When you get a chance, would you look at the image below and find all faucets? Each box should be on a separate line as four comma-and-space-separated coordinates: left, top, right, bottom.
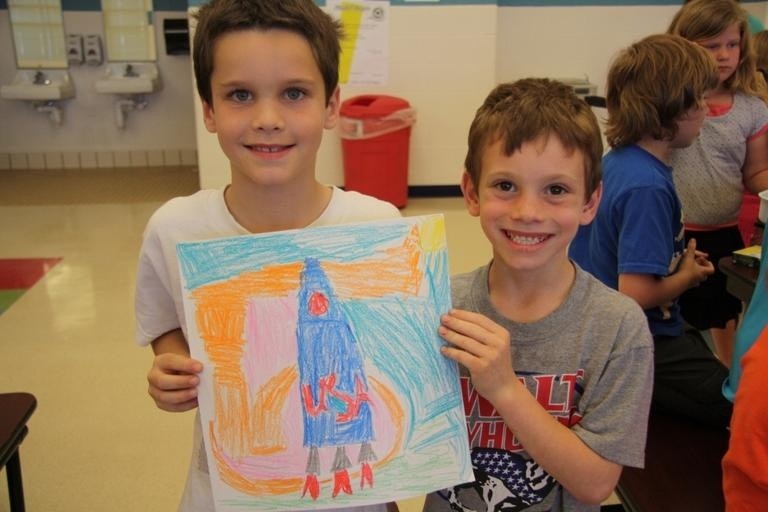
122, 63, 137, 76
32, 72, 43, 84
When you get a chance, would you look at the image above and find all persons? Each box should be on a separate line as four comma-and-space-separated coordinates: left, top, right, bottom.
419, 78, 655, 512
568, 32, 730, 497
662, 2, 767, 364
133, 2, 408, 512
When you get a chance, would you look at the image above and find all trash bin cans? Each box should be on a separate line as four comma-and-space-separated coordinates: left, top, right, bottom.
339, 95, 416, 209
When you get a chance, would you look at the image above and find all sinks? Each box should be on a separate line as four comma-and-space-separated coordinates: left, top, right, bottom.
95, 62, 163, 96
0, 70, 74, 102
